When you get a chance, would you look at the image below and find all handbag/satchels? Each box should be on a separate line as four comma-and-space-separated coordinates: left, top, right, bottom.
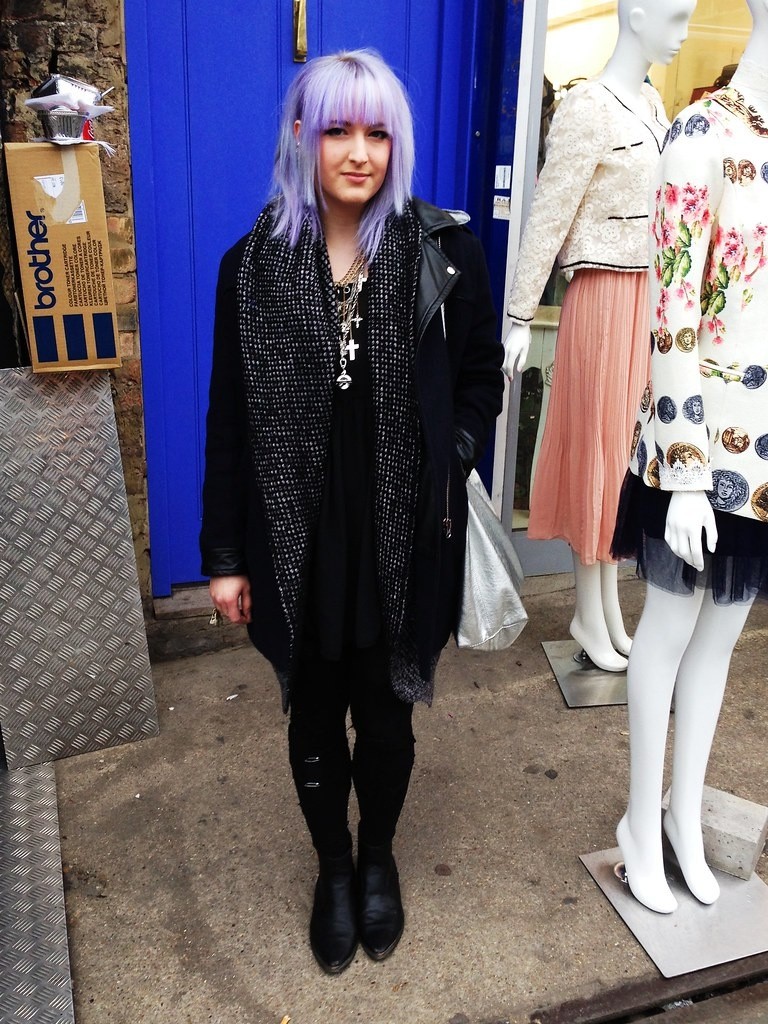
455, 470, 529, 655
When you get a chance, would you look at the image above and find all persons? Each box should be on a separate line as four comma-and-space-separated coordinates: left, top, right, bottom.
199, 48, 505, 975
502, 0, 697, 672
616, 0, 768, 914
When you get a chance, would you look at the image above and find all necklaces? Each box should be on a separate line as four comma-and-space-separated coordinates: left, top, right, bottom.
334, 249, 369, 391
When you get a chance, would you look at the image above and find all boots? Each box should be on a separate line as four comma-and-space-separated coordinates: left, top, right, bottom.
289, 724, 358, 974
350, 730, 415, 961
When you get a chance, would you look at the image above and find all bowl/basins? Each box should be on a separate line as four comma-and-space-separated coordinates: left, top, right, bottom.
33, 73, 101, 105
37, 109, 90, 141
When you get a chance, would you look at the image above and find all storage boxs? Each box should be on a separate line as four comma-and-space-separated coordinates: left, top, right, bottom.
4, 139, 124, 373
